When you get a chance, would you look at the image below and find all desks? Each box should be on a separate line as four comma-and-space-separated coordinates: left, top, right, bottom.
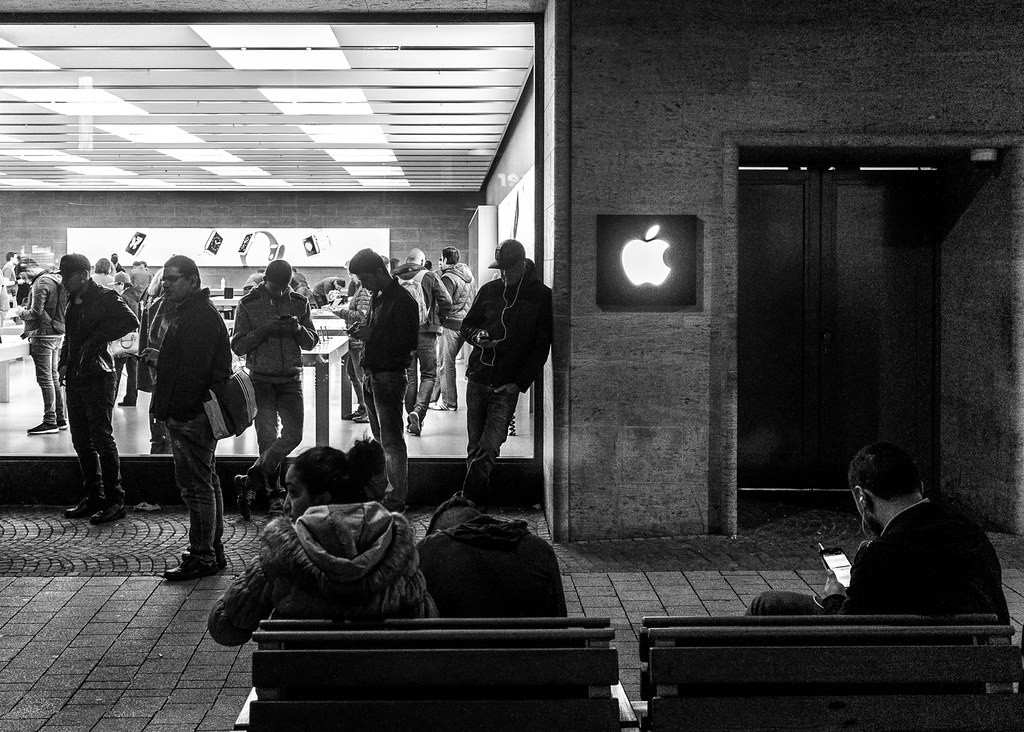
0, 289, 353, 448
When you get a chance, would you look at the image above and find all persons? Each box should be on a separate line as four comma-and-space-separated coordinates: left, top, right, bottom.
141, 255, 233, 581
349, 248, 420, 515
91, 253, 181, 454
0, 252, 67, 435
231, 260, 319, 521
328, 246, 477, 436
49, 253, 140, 524
208, 438, 438, 648
461, 239, 553, 513
744, 439, 1011, 627
243, 266, 345, 309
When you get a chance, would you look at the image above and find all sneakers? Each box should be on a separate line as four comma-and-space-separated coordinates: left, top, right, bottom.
57, 421, 68, 429
182, 551, 227, 567
428, 402, 437, 408
90, 504, 127, 525
234, 474, 255, 520
27, 423, 59, 434
433, 404, 456, 411
267, 498, 285, 521
164, 557, 219, 580
352, 413, 370, 423
65, 498, 107, 518
345, 409, 366, 419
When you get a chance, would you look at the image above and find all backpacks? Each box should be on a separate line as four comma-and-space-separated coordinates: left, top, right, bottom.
395, 270, 432, 325
35, 275, 67, 333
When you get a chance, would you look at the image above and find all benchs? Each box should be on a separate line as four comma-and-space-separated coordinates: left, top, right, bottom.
630, 611, 1024, 732
233, 615, 639, 732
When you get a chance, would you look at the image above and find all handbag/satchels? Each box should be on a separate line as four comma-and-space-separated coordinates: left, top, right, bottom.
107, 332, 139, 358
202, 370, 259, 440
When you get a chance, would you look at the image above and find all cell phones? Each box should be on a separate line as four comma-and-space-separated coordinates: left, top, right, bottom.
820, 548, 852, 590
125, 351, 153, 363
479, 336, 492, 341
343, 321, 359, 333
279, 314, 292, 320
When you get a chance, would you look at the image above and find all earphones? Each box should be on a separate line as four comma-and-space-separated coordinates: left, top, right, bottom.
859, 497, 866, 510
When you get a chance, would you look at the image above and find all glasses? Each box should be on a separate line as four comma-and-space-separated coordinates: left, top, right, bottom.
270, 285, 290, 292
162, 273, 184, 282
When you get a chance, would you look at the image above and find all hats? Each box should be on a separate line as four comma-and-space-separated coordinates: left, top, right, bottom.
49, 253, 91, 274
488, 239, 525, 269
107, 271, 131, 285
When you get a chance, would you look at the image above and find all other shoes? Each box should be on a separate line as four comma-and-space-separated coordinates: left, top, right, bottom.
409, 412, 421, 436
381, 502, 403, 513
118, 402, 137, 408
